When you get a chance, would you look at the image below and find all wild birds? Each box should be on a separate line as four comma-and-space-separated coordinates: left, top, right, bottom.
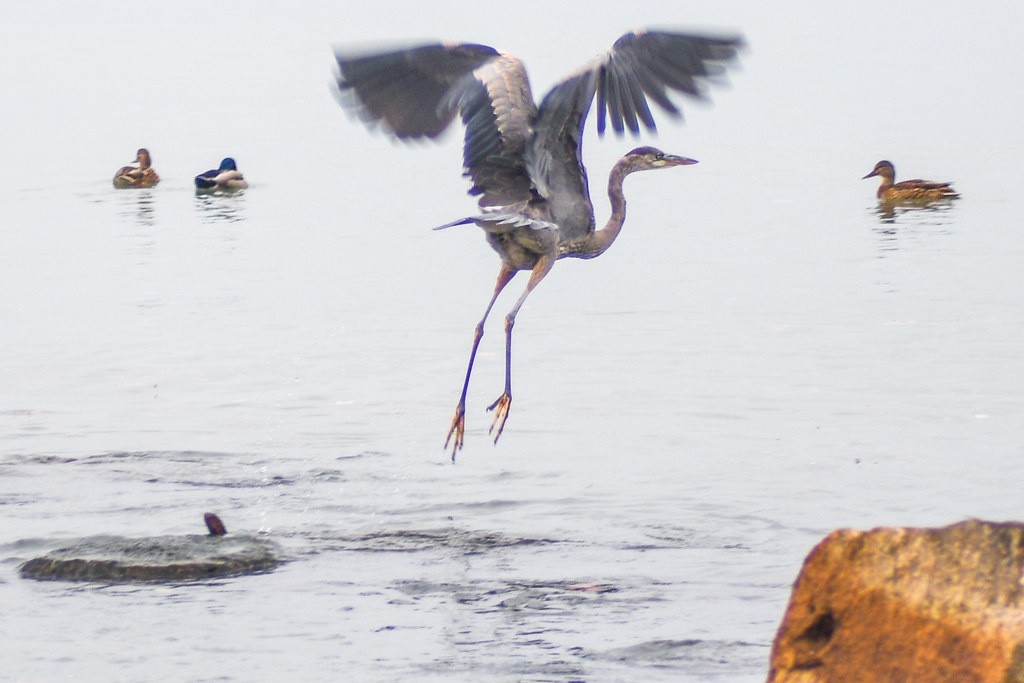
317, 23, 755, 465
194, 157, 249, 196
861, 159, 962, 204
112, 148, 160, 190
